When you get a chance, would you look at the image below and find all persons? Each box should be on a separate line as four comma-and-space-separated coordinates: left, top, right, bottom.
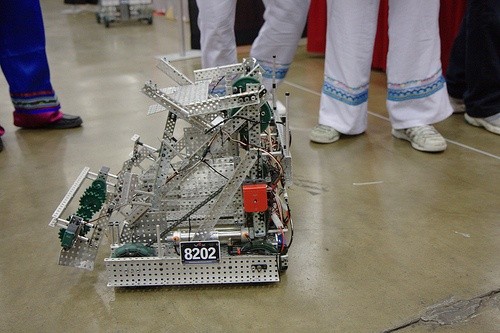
309, 0, 453, 152
0, 0, 82, 151
195, 0, 310, 125
445, 0, 500, 135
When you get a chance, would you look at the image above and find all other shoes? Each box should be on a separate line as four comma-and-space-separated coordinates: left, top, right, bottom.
22, 112, 83, 130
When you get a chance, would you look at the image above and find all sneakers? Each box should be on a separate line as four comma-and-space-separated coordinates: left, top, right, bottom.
449, 96, 465, 113
309, 124, 340, 144
464, 112, 500, 135
391, 124, 447, 153
